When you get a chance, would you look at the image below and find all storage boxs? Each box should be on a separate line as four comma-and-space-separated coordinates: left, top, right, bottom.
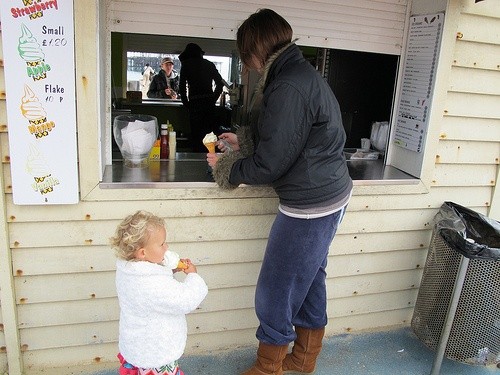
343, 147, 380, 161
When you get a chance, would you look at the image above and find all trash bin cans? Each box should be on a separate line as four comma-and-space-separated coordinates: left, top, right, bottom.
410, 201, 500, 367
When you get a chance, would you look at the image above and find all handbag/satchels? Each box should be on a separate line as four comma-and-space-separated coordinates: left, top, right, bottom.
142, 72, 144, 75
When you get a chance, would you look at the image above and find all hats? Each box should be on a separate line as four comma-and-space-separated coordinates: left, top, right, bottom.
161, 57, 174, 64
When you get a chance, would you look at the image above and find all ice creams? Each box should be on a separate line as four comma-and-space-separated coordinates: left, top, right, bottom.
202, 131, 217, 153
163, 250, 188, 269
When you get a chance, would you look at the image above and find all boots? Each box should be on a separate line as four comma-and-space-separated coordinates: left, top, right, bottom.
282, 326, 325, 374
240, 342, 289, 375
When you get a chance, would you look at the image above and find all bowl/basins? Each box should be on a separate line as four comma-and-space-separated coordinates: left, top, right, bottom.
113, 114, 158, 169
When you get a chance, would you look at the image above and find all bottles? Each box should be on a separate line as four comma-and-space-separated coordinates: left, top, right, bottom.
161, 120, 176, 160
220, 91, 226, 107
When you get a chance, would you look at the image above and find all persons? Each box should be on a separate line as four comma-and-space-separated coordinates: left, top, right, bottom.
206, 9, 355, 375
110, 210, 208, 375
178, 43, 225, 152
147, 57, 180, 146
142, 63, 155, 87
